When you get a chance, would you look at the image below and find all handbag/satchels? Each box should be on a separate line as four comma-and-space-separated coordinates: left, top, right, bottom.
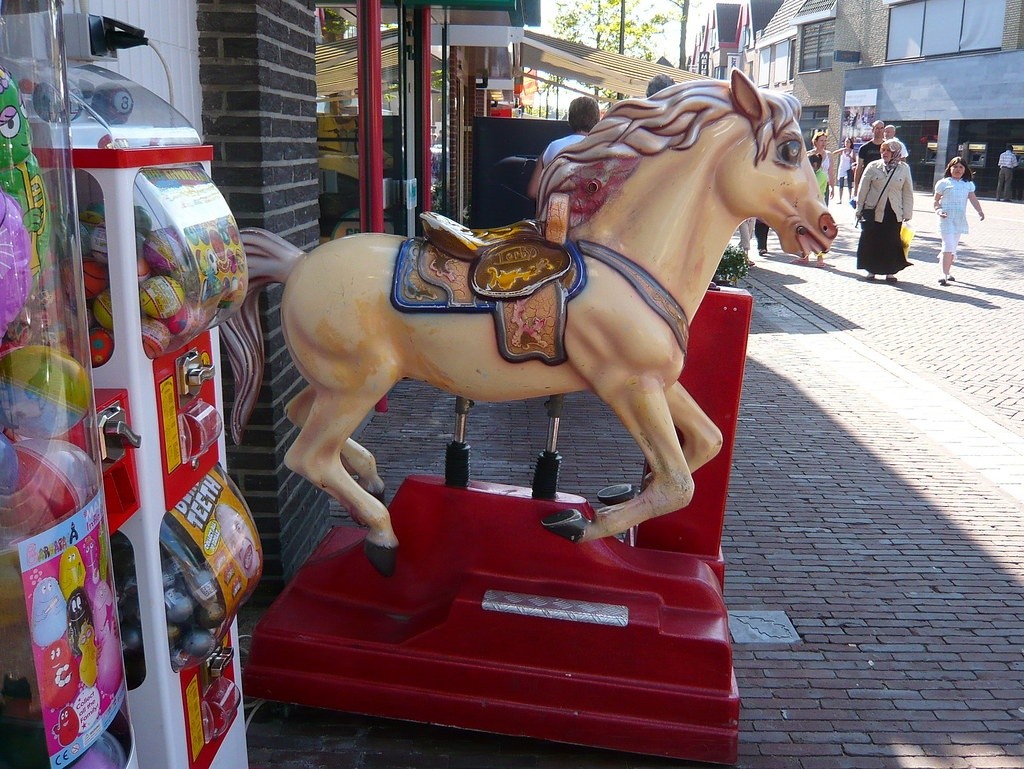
860, 209, 875, 231
847, 169, 853, 183
900, 219, 916, 262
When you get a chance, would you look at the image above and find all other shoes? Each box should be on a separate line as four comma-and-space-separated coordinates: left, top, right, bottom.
866, 275, 874, 280
790, 257, 808, 265
759, 249, 766, 256
997, 198, 1000, 200
748, 260, 754, 266
947, 276, 954, 281
939, 279, 946, 285
1003, 198, 1012, 202
886, 276, 897, 282
816, 262, 825, 268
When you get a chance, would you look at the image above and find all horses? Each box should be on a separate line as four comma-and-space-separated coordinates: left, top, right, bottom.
219, 66, 838, 579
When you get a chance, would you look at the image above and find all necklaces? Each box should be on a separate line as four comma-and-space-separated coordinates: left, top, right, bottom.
854, 139, 913, 282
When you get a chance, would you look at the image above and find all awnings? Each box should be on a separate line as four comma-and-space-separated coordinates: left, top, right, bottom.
515, 29, 720, 104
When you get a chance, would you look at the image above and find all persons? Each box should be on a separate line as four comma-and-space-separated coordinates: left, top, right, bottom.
529, 96, 601, 192
805, 132, 835, 207
854, 120, 887, 197
790, 153, 827, 267
993, 144, 1018, 201
933, 156, 984, 283
884, 125, 908, 163
754, 216, 769, 256
739, 217, 757, 267
833, 136, 855, 204
